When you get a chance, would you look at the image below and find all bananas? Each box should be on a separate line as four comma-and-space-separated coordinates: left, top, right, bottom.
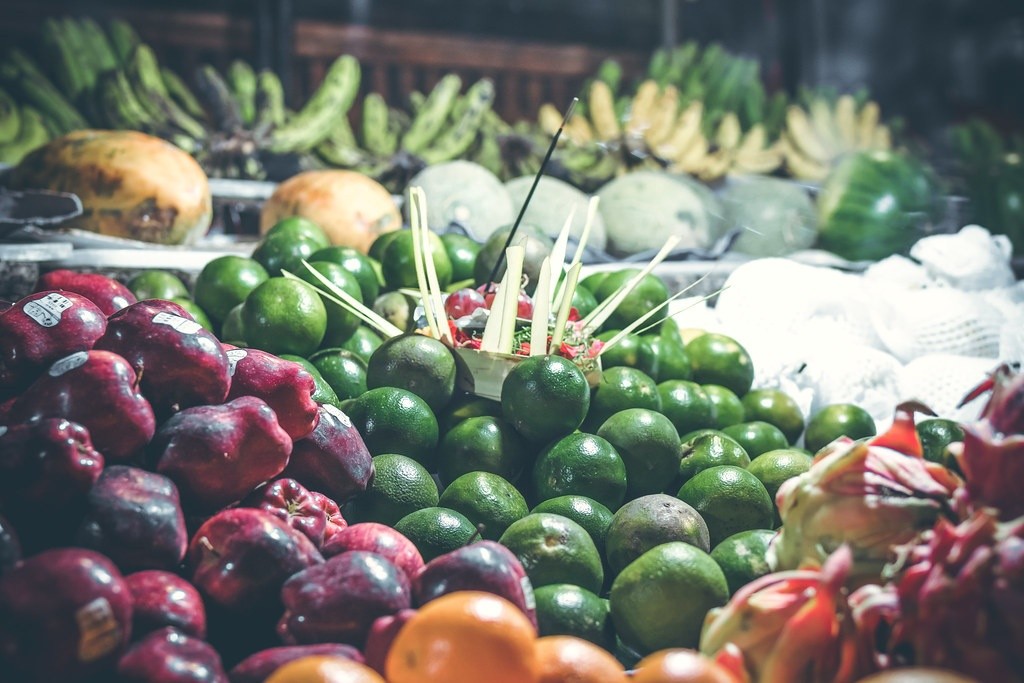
0, 0, 1024, 192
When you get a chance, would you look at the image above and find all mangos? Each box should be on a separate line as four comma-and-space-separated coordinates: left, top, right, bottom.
17, 132, 818, 264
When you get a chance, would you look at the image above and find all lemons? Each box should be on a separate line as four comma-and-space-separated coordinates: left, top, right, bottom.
128, 219, 975, 656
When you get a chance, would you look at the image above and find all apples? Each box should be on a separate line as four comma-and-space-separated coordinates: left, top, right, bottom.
0, 267, 537, 683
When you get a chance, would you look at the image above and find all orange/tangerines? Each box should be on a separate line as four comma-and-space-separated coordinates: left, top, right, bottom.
266, 590, 743, 683
